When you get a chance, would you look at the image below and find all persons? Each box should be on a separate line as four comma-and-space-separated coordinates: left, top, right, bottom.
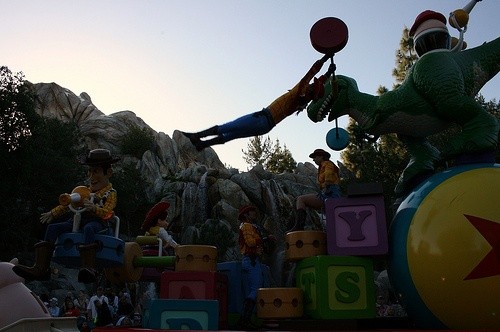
142, 201, 180, 257
376, 295, 407, 318
284, 149, 342, 232
12, 148, 117, 283
183, 53, 336, 151
40, 286, 141, 332
237, 203, 277, 322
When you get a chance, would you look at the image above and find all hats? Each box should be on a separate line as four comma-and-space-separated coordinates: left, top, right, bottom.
49, 297, 59, 305
